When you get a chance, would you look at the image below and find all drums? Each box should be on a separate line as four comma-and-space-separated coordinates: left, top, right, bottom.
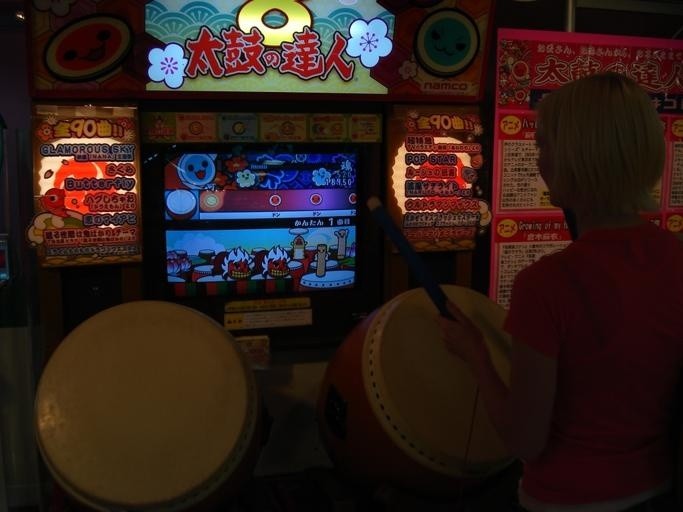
35, 301, 264, 511
318, 286, 516, 493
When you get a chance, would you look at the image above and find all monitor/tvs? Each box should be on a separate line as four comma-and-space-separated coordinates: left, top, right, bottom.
153, 142, 358, 298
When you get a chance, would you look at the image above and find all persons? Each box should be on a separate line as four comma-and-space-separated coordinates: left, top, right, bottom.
434, 72, 683, 510
